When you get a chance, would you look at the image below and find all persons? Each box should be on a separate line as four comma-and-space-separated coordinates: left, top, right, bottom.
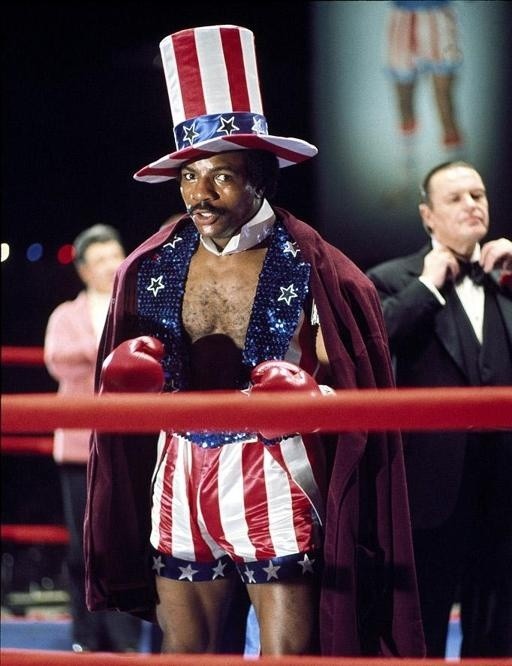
45, 223, 147, 658
380, 1, 471, 198
365, 159, 512, 659
80, 147, 427, 661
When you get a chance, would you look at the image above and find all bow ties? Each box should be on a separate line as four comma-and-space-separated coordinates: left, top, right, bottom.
447, 256, 492, 290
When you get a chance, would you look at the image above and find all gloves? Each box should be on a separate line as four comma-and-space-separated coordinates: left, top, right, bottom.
248, 360, 339, 441
100, 333, 166, 397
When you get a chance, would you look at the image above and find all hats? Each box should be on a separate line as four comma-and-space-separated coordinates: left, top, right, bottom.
131, 20, 320, 183
